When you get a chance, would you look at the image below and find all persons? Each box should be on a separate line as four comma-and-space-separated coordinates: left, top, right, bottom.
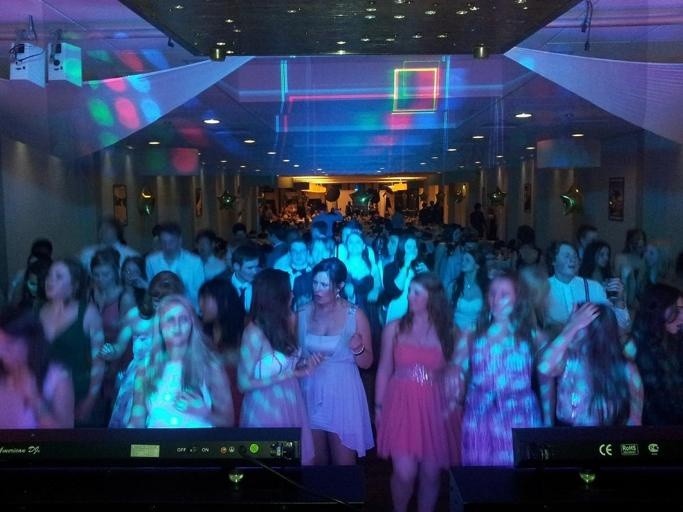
1, 200, 683, 512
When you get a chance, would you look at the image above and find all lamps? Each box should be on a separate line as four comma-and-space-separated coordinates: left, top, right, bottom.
209, 45, 226, 61
471, 40, 490, 59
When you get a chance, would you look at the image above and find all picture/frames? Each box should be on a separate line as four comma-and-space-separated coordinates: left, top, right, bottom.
607, 176, 624, 221
111, 184, 128, 227
